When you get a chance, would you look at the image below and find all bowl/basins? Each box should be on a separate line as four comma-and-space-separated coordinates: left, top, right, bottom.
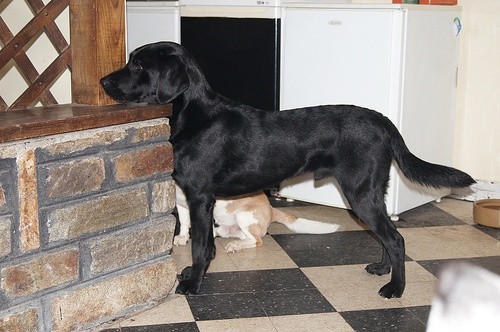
473, 199, 500, 228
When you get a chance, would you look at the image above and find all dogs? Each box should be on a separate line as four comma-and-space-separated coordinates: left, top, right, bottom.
99, 41, 478, 299
173, 181, 341, 254
